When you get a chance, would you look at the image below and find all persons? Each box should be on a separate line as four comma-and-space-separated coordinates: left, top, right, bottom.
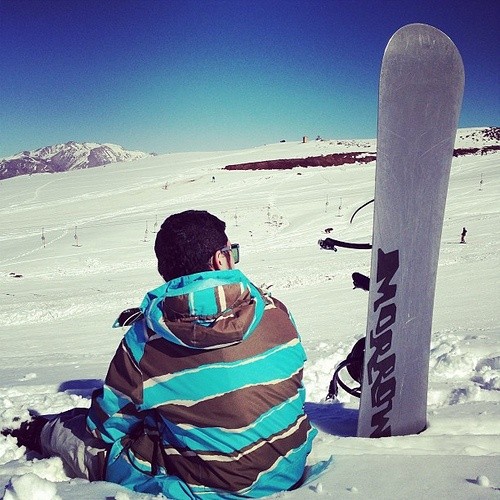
460, 227, 467, 243
1, 210, 318, 500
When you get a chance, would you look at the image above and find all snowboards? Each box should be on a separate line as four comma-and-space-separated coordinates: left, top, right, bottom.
357, 24, 465, 441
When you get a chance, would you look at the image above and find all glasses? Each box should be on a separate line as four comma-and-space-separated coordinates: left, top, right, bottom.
220, 244, 240, 264
